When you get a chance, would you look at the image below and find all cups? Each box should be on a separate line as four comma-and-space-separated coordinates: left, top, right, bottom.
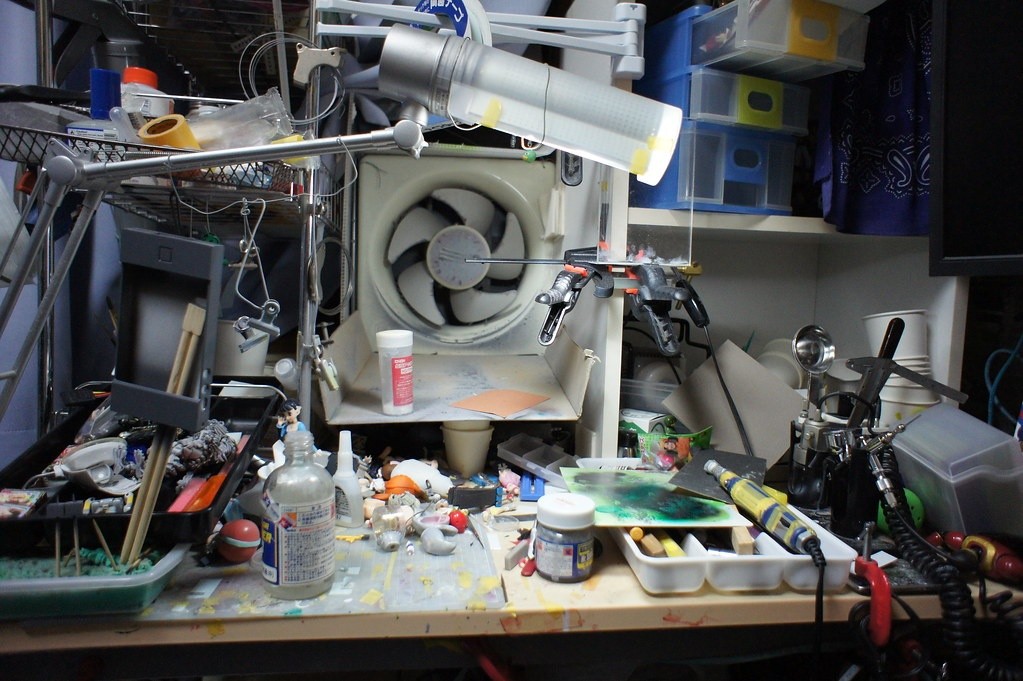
212, 319, 269, 376
441, 426, 495, 478
753, 308, 940, 430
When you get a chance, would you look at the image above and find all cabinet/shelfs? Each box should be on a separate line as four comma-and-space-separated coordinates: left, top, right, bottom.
0, 1, 1023, 681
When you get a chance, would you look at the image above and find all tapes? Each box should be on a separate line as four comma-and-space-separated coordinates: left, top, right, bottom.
139, 113, 200, 152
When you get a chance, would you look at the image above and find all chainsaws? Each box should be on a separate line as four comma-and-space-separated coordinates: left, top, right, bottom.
465, 247, 709, 361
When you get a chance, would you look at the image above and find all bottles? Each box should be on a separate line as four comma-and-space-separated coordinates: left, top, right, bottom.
332, 431, 363, 528
375, 329, 414, 415
119, 66, 175, 117
274, 358, 299, 390
259, 431, 335, 599
533, 493, 595, 582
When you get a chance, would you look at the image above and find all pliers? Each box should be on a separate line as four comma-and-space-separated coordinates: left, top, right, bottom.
537, 271, 581, 347
631, 265, 681, 356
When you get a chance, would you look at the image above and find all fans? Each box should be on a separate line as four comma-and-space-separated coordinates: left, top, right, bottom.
353, 146, 559, 355
